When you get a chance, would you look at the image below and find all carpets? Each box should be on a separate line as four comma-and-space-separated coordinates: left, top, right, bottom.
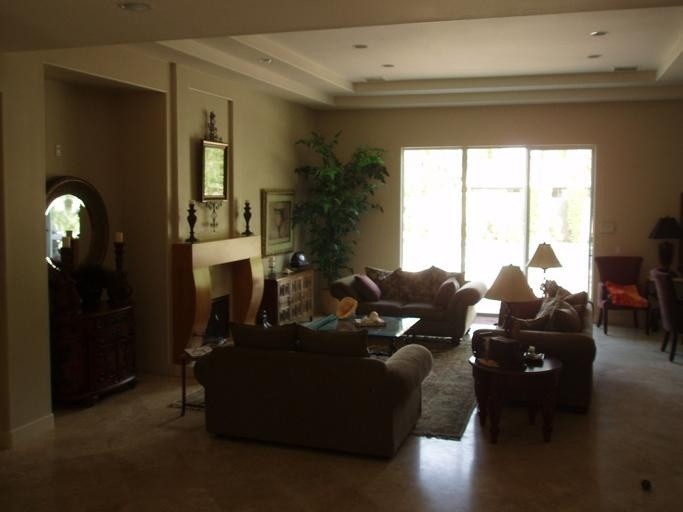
170, 333, 478, 441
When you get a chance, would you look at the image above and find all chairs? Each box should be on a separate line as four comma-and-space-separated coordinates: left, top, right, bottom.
594, 256, 682, 361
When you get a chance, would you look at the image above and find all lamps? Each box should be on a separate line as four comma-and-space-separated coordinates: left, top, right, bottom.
648, 215, 683, 277
484, 242, 562, 364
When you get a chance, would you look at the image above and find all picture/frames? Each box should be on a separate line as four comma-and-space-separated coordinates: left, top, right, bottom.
201, 139, 228, 204
260, 188, 295, 259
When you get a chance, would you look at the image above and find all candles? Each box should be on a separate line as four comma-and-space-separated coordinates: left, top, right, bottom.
245, 200, 249, 203
62, 236, 70, 247
190, 200, 195, 204
116, 231, 123, 242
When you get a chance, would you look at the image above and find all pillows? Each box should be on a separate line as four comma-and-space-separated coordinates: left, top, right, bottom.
354, 266, 465, 311
503, 280, 588, 333
226, 319, 370, 357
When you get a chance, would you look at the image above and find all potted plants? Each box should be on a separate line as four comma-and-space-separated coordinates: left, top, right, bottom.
292, 127, 389, 315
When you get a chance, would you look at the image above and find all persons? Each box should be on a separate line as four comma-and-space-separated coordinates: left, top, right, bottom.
269, 206, 288, 239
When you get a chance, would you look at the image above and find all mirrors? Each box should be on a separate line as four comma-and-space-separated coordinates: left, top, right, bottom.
46, 175, 110, 284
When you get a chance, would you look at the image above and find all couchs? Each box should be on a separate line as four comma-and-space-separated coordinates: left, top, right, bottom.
192, 341, 433, 459
472, 297, 597, 416
329, 271, 484, 343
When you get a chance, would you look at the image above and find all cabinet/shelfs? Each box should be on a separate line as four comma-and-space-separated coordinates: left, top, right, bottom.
257, 267, 314, 326
50, 300, 138, 406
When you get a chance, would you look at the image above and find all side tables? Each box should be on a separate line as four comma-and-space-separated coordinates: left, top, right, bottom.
468, 353, 564, 443
180, 340, 225, 417
